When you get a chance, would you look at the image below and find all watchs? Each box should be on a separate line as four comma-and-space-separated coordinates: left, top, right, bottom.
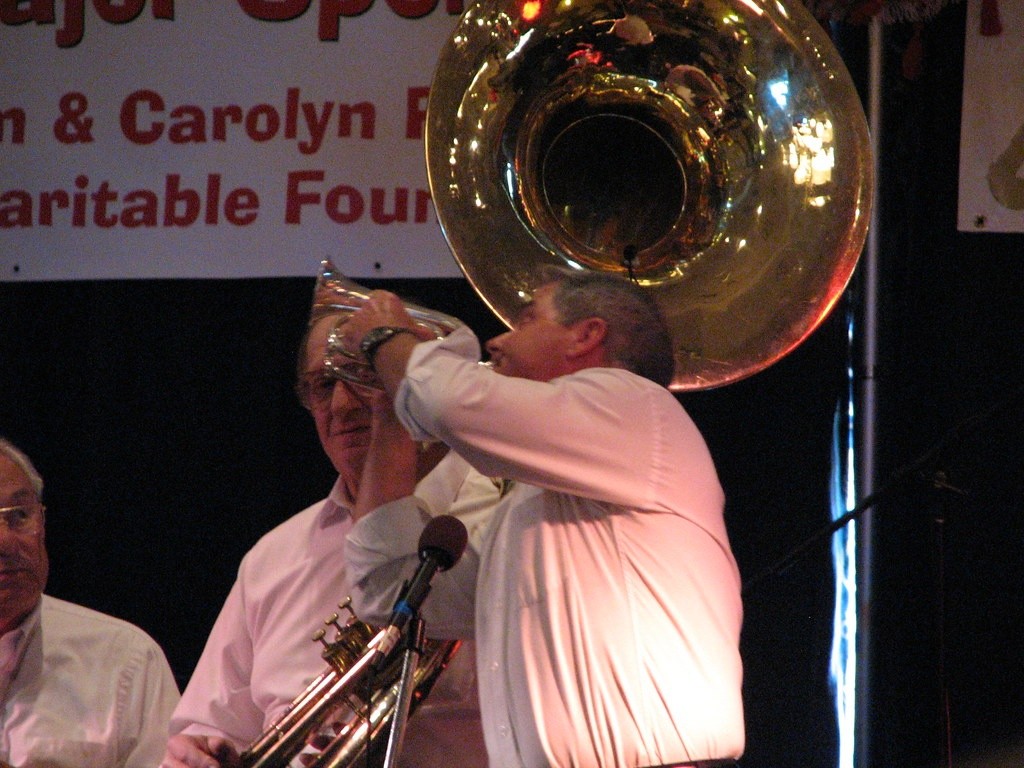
358, 325, 420, 373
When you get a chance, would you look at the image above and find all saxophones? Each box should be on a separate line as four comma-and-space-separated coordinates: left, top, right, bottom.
226, 593, 466, 768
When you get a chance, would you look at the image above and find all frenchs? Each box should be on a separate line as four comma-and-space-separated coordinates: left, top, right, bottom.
304, 1, 877, 391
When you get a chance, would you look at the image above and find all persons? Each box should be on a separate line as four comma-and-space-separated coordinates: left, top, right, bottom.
2, 441, 182, 768
343, 268, 747, 768
155, 280, 504, 768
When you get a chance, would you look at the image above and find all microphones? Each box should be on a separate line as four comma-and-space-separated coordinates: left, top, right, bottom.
371, 515, 468, 669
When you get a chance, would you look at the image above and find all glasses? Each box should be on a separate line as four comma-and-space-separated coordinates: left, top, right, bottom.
296, 359, 391, 410
0, 502, 46, 538
